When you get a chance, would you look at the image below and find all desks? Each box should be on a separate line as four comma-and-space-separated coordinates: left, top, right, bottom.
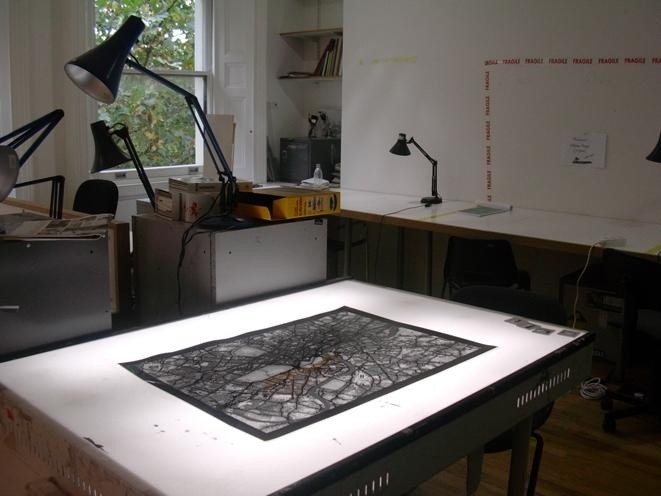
2, 274, 596, 496
132, 213, 329, 326
330, 185, 661, 268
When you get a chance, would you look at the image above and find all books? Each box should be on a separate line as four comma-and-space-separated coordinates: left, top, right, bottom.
278, 34, 344, 77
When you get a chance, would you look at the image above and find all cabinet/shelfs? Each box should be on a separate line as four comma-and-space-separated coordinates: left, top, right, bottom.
277, 27, 343, 81
279, 138, 340, 184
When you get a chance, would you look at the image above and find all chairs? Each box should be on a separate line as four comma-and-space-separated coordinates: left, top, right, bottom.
442, 237, 659, 493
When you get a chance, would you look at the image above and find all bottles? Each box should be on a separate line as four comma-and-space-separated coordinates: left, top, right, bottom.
314, 164, 323, 187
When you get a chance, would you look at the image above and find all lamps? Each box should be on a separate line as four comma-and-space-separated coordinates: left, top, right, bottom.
390, 132, 444, 205
63, 15, 248, 232
0, 108, 65, 220
